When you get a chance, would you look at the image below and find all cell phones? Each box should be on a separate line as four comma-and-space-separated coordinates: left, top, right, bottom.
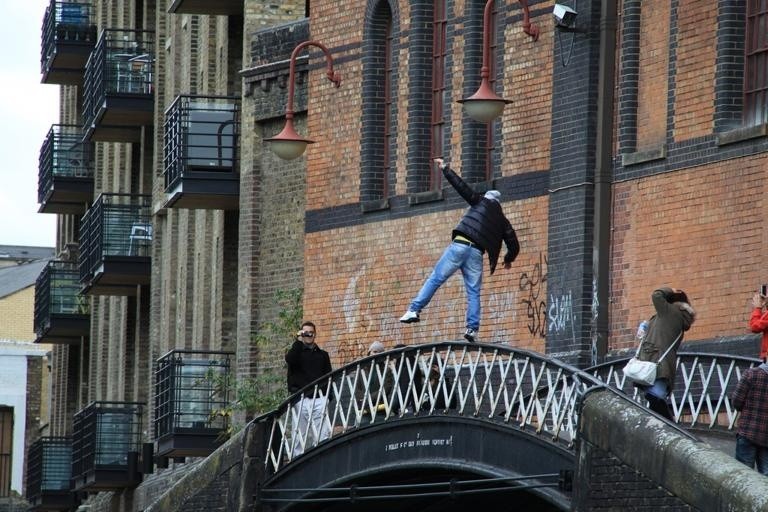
760, 285, 768, 297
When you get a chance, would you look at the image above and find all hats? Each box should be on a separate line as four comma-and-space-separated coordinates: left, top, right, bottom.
484, 189, 501, 202
366, 341, 386, 354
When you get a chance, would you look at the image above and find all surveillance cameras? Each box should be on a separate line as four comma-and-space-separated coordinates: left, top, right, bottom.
552, 3, 578, 28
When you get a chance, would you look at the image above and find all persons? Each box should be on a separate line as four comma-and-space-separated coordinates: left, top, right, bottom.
389, 344, 420, 414
634, 287, 693, 424
730, 357, 767, 478
748, 291, 767, 366
284, 322, 332, 460
399, 157, 520, 344
422, 356, 456, 412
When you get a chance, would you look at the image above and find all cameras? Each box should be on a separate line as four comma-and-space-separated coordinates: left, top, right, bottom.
301, 332, 314, 337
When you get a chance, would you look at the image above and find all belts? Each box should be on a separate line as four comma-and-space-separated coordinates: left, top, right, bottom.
452, 238, 489, 256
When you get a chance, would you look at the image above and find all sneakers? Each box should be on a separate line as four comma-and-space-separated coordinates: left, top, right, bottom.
399, 310, 419, 324
464, 329, 476, 342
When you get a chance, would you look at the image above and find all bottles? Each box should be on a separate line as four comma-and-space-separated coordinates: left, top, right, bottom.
636, 319, 649, 339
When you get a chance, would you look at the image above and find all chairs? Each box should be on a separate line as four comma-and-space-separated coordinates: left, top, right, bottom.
124, 51, 154, 94
125, 219, 155, 257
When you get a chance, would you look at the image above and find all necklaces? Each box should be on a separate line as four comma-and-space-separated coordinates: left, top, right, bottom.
354, 341, 394, 427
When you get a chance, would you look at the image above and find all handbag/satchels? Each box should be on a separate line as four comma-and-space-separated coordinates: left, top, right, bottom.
622, 353, 660, 387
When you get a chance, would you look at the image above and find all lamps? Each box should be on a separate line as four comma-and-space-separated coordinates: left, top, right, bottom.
454, 0, 542, 126
261, 38, 340, 160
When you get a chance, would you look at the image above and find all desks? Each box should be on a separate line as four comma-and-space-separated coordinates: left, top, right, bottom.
112, 48, 148, 95
58, 283, 84, 315
